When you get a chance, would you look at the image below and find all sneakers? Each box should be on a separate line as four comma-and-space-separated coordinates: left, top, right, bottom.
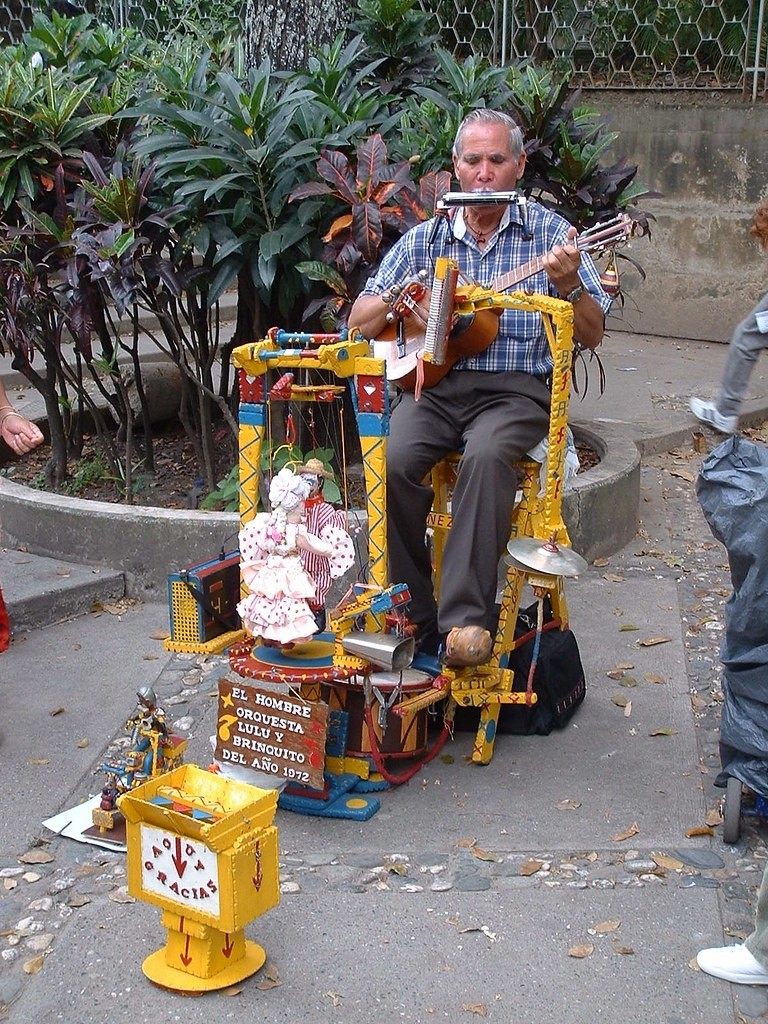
697, 944, 768, 984
689, 396, 738, 434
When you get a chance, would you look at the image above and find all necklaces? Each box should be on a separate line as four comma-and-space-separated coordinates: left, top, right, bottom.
463, 215, 499, 243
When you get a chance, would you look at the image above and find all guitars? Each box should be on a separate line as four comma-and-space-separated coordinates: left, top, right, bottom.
373, 213, 632, 392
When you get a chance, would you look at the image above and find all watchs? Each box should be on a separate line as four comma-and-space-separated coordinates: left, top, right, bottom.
559, 282, 584, 302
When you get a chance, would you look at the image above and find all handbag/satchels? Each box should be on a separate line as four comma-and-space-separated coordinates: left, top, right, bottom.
419, 596, 586, 735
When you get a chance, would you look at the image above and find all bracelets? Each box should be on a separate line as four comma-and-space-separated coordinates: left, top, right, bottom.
0, 405, 16, 412
0, 412, 24, 436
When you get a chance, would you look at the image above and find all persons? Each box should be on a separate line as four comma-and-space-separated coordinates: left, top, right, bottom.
0, 381, 45, 652
347, 108, 613, 668
236, 458, 355, 650
695, 856, 768, 984
689, 197, 768, 435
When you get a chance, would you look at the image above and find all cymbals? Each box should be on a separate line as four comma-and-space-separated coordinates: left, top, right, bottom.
503, 536, 588, 576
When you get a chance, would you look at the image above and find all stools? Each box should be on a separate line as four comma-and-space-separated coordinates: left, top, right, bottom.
430, 450, 569, 765
